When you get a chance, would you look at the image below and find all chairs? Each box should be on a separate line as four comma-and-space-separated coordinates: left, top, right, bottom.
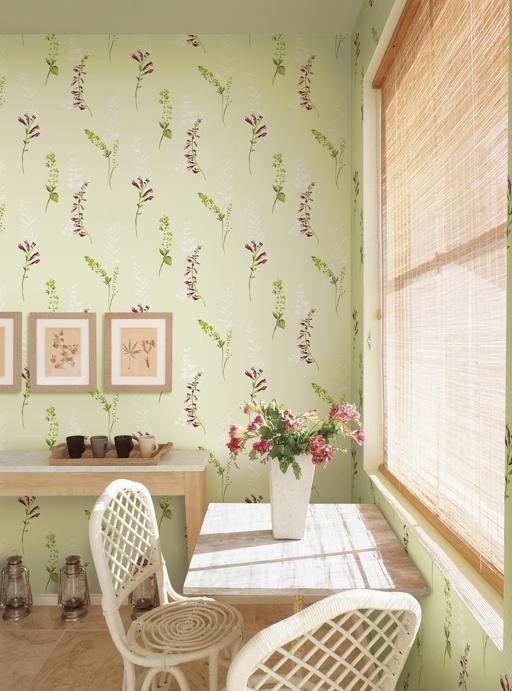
89, 478, 246, 691
226, 585, 424, 691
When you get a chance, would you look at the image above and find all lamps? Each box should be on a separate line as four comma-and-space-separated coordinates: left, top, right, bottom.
127, 557, 158, 622
0, 556, 35, 625
56, 555, 91, 625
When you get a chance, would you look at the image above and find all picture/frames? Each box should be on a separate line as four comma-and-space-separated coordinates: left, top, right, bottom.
0, 311, 24, 397
102, 310, 174, 396
27, 311, 99, 396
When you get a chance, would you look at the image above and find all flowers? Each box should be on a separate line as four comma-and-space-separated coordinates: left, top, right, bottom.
226, 398, 367, 478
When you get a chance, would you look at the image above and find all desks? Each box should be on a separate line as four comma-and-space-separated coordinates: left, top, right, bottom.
1, 448, 211, 566
181, 500, 435, 691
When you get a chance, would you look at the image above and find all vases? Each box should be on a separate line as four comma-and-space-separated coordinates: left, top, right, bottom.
267, 454, 316, 542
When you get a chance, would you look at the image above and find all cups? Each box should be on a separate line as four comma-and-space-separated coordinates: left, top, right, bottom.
137, 434, 159, 459
90, 435, 110, 458
65, 435, 86, 458
113, 434, 134, 458
